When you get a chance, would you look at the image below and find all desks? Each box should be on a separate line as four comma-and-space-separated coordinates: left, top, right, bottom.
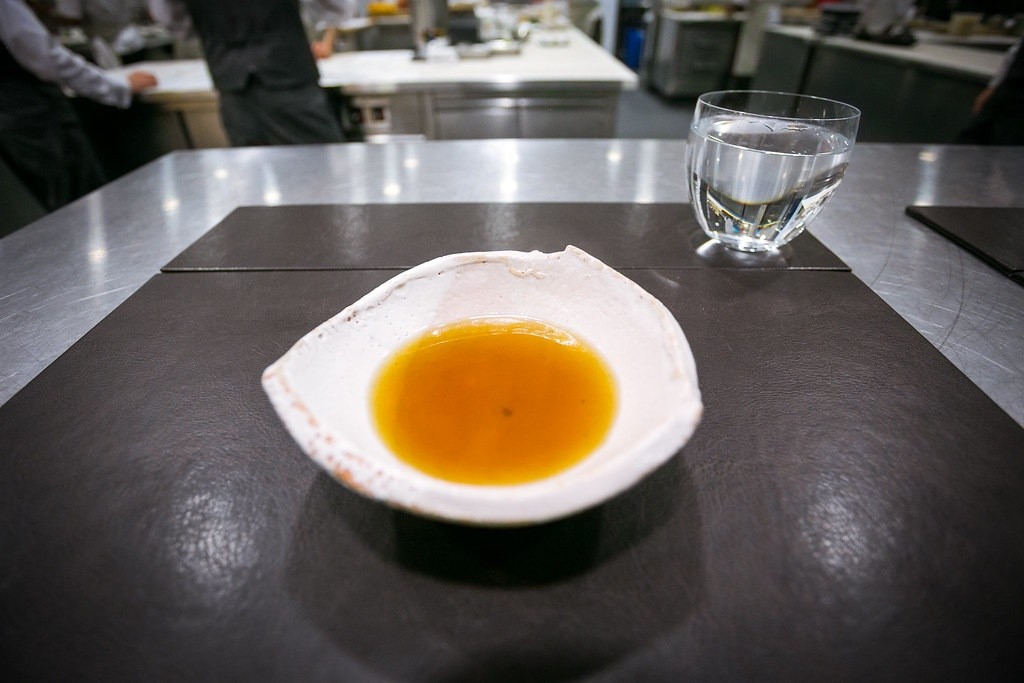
0, 138, 1024, 683
111, 14, 639, 152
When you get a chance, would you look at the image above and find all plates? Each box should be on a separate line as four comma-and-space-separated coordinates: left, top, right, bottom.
261, 244, 703, 527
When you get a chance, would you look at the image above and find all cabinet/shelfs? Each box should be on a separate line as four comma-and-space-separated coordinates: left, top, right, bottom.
637, 6, 1024, 143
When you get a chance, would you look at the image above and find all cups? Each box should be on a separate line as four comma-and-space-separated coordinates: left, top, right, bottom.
685, 90, 861, 268
542, 1, 570, 49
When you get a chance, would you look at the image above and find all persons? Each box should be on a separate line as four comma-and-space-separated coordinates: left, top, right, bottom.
148, 0, 344, 147
1, 0, 156, 213
